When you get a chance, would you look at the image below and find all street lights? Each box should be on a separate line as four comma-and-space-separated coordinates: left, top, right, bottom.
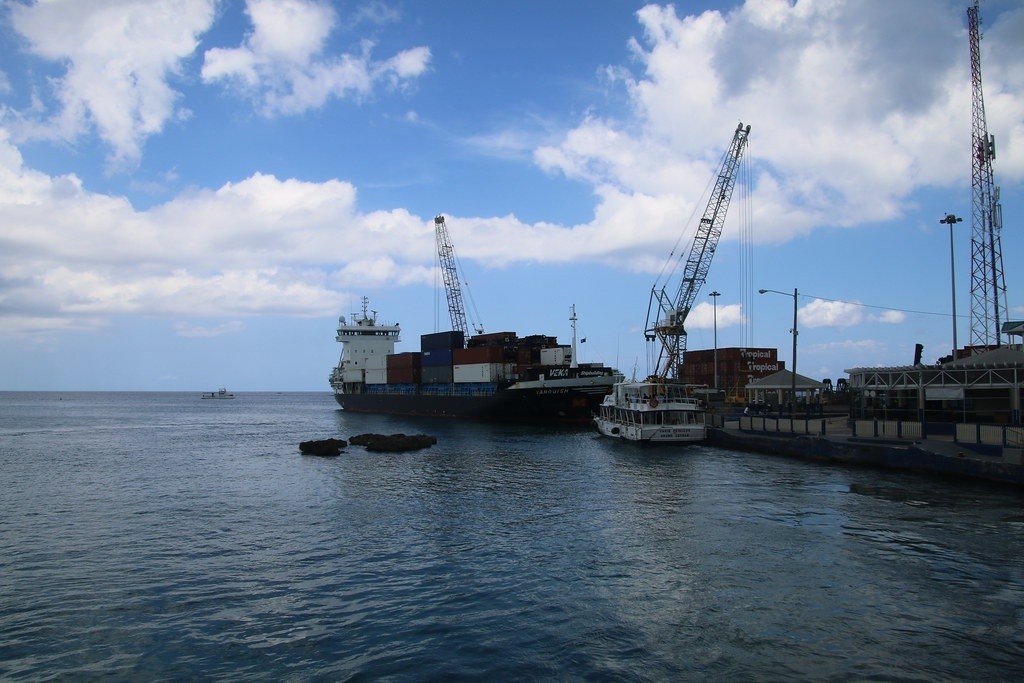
938, 211, 965, 366
707, 290, 721, 393
757, 290, 801, 415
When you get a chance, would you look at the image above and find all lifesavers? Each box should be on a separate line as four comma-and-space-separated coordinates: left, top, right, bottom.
650, 398, 659, 408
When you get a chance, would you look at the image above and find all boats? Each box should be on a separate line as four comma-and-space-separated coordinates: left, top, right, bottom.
327, 215, 625, 421
589, 374, 708, 444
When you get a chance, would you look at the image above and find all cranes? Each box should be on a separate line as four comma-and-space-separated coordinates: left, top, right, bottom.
641, 120, 752, 387
961, 3, 1016, 362
